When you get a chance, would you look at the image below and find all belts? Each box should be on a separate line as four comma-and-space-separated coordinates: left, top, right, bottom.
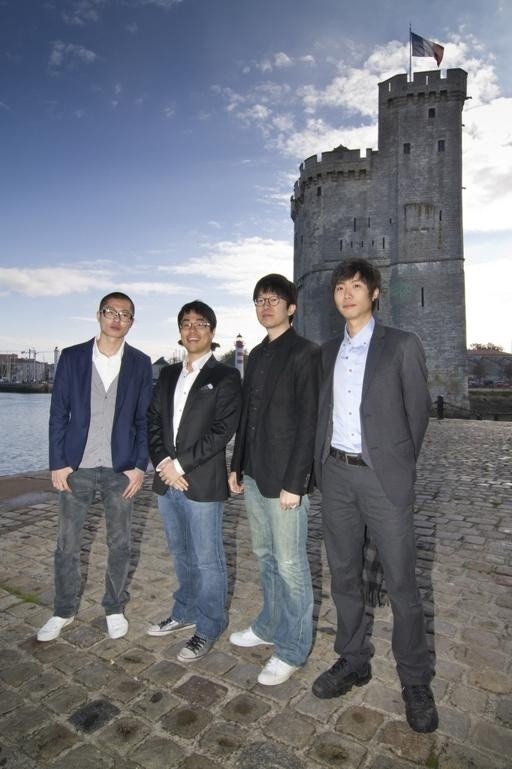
330, 446, 366, 466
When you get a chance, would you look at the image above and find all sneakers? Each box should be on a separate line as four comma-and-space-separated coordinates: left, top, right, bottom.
37, 616, 74, 642
105, 613, 129, 639
177, 634, 214, 663
229, 626, 275, 647
258, 654, 303, 686
145, 616, 196, 636
312, 657, 372, 699
402, 683, 439, 732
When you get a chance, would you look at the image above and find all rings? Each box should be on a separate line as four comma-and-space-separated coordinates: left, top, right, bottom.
291, 504, 298, 509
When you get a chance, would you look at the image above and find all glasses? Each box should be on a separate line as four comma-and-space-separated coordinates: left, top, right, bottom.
99, 308, 133, 320
178, 320, 212, 329
254, 296, 280, 307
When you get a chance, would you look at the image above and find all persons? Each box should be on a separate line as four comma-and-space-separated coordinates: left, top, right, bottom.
228, 274, 322, 685
146, 301, 244, 662
313, 256, 440, 732
35, 292, 153, 643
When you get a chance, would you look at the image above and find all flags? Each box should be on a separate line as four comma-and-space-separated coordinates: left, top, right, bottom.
411, 32, 445, 67
